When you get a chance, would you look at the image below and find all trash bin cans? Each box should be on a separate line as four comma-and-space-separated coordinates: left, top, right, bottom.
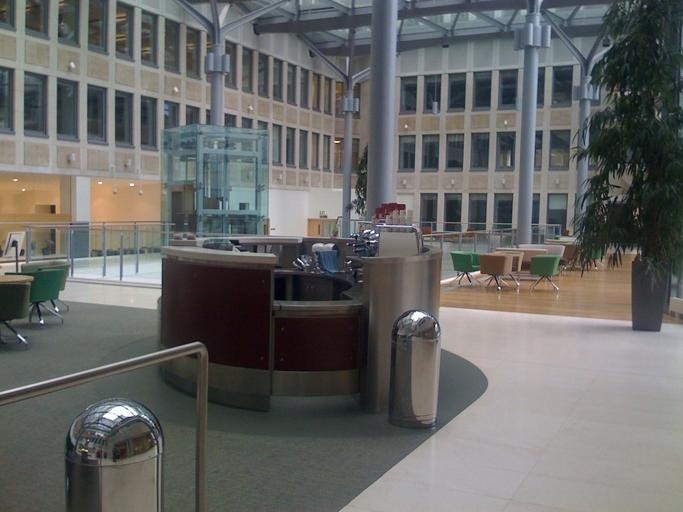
65, 397, 164, 512
388, 309, 442, 428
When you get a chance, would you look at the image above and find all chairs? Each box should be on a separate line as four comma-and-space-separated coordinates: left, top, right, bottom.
0, 257, 71, 347
450, 238, 577, 293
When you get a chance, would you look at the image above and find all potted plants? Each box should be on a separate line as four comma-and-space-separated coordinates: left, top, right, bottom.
567, 0, 683, 331
